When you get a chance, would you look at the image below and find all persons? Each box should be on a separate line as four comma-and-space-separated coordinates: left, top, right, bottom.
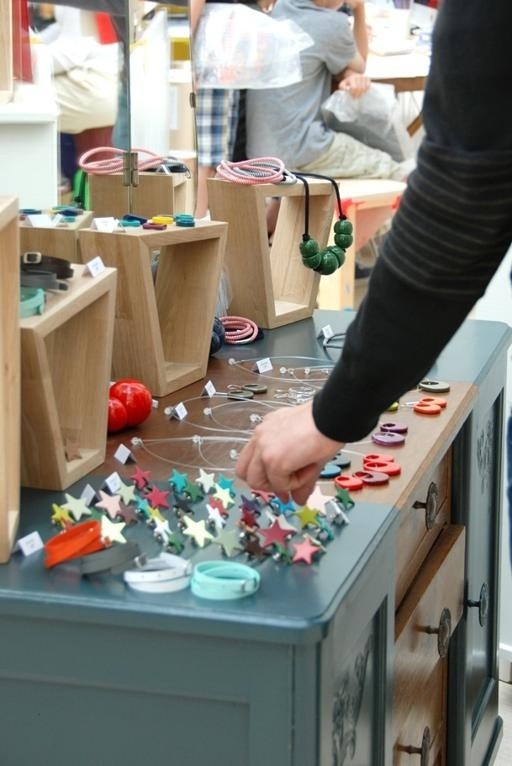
191, 0, 276, 219
245, 1, 408, 178
237, 1, 511, 512
32, 2, 123, 189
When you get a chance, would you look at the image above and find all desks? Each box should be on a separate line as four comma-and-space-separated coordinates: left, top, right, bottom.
0, 310, 511, 765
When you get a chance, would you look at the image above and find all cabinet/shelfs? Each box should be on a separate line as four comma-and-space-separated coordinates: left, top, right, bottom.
0, 175, 340, 491
